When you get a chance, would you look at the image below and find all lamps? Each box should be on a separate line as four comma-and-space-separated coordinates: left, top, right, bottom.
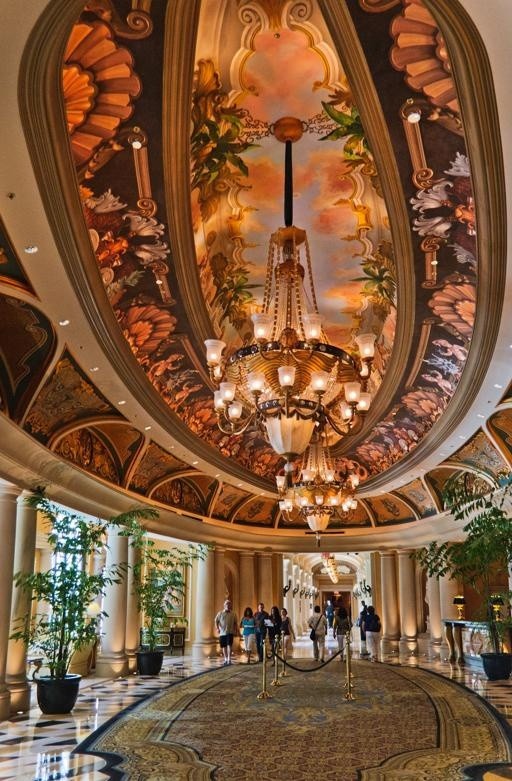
351, 577, 372, 600
203, 113, 375, 478
320, 553, 342, 587
282, 575, 322, 600
452, 595, 466, 621
490, 595, 506, 623
276, 440, 360, 550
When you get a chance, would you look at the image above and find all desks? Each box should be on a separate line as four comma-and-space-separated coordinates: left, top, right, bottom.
140, 628, 186, 656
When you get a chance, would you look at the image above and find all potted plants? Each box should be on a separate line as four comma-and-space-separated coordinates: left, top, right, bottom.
8, 482, 159, 717
406, 468, 512, 683
119, 544, 206, 680
174, 617, 189, 629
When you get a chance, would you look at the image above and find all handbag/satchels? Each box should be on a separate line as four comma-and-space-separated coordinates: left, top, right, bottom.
311, 629, 316, 640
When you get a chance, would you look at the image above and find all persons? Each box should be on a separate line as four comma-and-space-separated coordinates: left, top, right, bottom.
325, 600, 335, 629
358, 600, 382, 663
306, 606, 329, 663
213, 599, 295, 665
333, 607, 353, 662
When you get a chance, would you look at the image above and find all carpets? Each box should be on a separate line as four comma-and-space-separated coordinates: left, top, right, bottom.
69, 656, 512, 781
